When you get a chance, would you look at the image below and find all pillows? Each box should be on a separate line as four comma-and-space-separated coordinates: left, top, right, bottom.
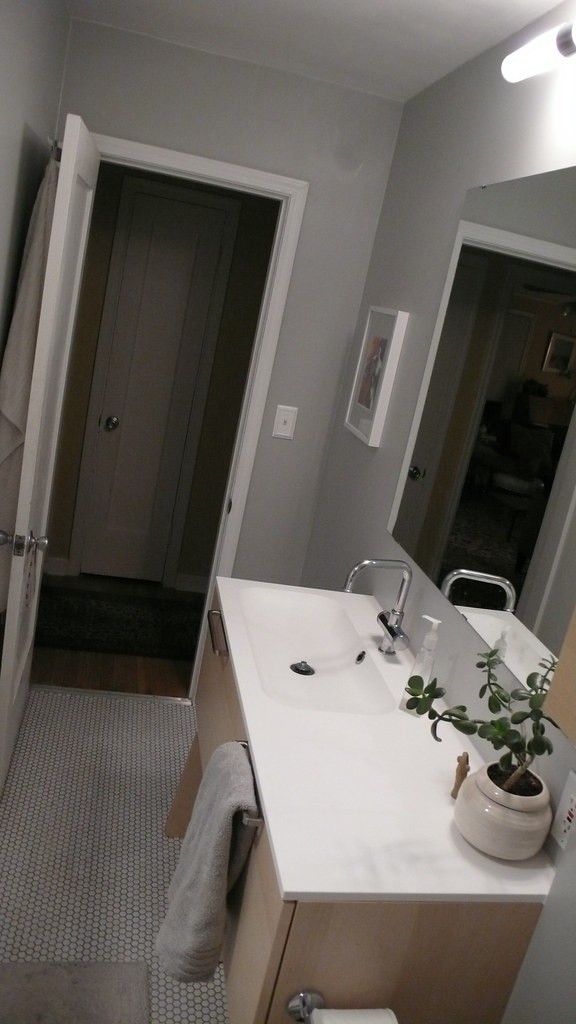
509, 422, 554, 470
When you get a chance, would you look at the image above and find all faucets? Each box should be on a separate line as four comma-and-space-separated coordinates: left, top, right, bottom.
439, 567, 518, 613
341, 558, 414, 656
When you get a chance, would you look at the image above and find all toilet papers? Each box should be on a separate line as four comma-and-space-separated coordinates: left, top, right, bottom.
308, 1007, 399, 1024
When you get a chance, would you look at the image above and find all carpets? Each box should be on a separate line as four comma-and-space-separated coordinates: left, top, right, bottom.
0, 961, 150, 1024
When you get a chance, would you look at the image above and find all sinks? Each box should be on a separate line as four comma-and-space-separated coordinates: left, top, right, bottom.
232, 579, 396, 716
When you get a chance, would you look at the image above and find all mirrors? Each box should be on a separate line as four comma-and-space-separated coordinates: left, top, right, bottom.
387, 166, 576, 747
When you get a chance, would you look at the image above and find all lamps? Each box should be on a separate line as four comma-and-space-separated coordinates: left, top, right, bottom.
497, 19, 576, 83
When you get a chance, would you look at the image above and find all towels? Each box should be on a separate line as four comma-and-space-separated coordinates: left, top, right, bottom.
0, 157, 61, 613
153, 741, 260, 985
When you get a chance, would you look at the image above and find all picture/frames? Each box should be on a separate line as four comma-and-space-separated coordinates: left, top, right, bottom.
344, 305, 409, 448
541, 332, 576, 375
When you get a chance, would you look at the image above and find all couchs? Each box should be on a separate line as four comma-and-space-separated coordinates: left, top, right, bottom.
464, 403, 570, 499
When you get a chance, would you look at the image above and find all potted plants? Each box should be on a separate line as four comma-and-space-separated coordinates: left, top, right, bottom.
403, 655, 562, 861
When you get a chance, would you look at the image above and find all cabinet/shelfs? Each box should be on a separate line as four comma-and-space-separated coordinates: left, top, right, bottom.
164, 584, 544, 1024
